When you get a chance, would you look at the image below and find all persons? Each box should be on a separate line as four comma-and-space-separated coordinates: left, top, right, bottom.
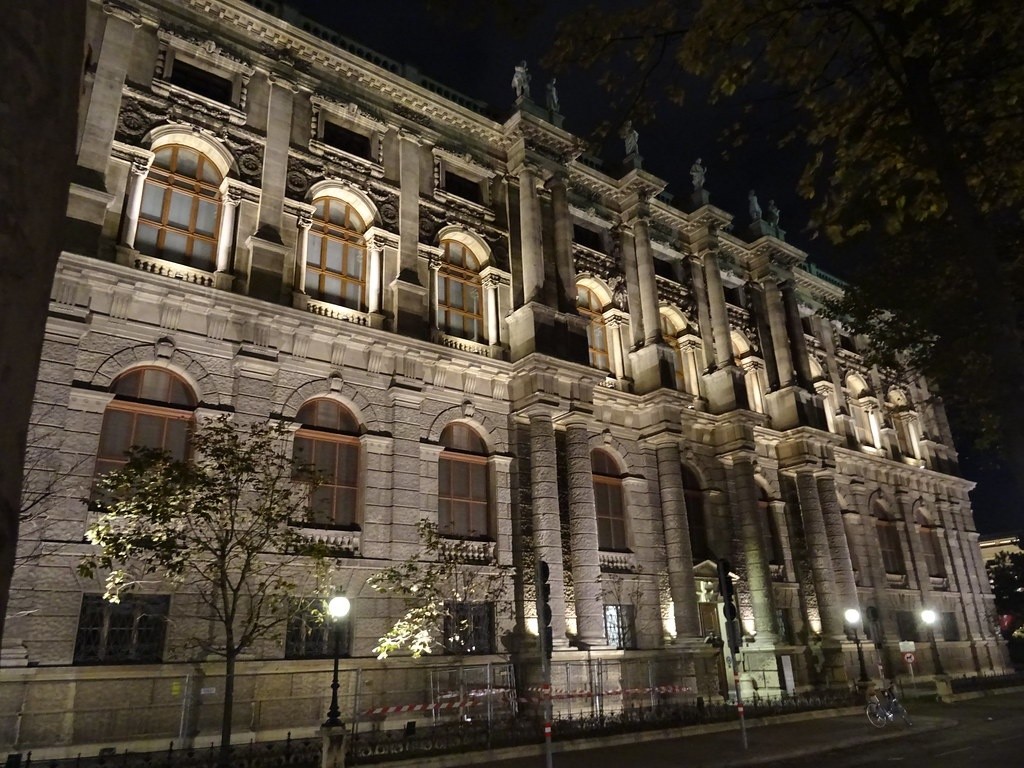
546, 77, 560, 112
619, 120, 639, 155
748, 190, 762, 218
511, 60, 531, 97
689, 157, 707, 190
768, 200, 781, 226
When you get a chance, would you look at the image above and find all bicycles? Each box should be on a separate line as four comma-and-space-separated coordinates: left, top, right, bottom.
864, 681, 914, 729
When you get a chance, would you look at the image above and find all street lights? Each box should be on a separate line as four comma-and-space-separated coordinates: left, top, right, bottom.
921, 608, 946, 674
844, 608, 871, 682
321, 596, 352, 728
